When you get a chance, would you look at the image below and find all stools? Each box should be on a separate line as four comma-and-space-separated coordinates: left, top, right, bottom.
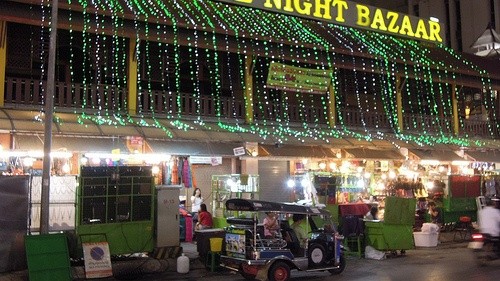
206, 250, 222, 272
344, 230, 367, 258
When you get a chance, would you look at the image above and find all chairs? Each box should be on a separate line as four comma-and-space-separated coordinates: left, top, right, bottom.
279, 218, 305, 258
253, 224, 275, 241
452, 214, 473, 244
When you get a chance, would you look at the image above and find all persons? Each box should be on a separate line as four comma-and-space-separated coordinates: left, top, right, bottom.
263, 211, 283, 238
477, 196, 500, 236
365, 206, 379, 220
191, 187, 204, 240
194, 203, 213, 230
427, 202, 442, 225
290, 213, 310, 248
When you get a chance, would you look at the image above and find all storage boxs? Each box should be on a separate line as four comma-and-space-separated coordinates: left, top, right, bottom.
411, 221, 441, 249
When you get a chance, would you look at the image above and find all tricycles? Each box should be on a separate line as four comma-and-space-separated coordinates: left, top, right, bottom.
216, 197, 347, 281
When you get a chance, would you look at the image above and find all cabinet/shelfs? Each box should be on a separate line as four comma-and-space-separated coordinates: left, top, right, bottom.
75, 166, 155, 256
